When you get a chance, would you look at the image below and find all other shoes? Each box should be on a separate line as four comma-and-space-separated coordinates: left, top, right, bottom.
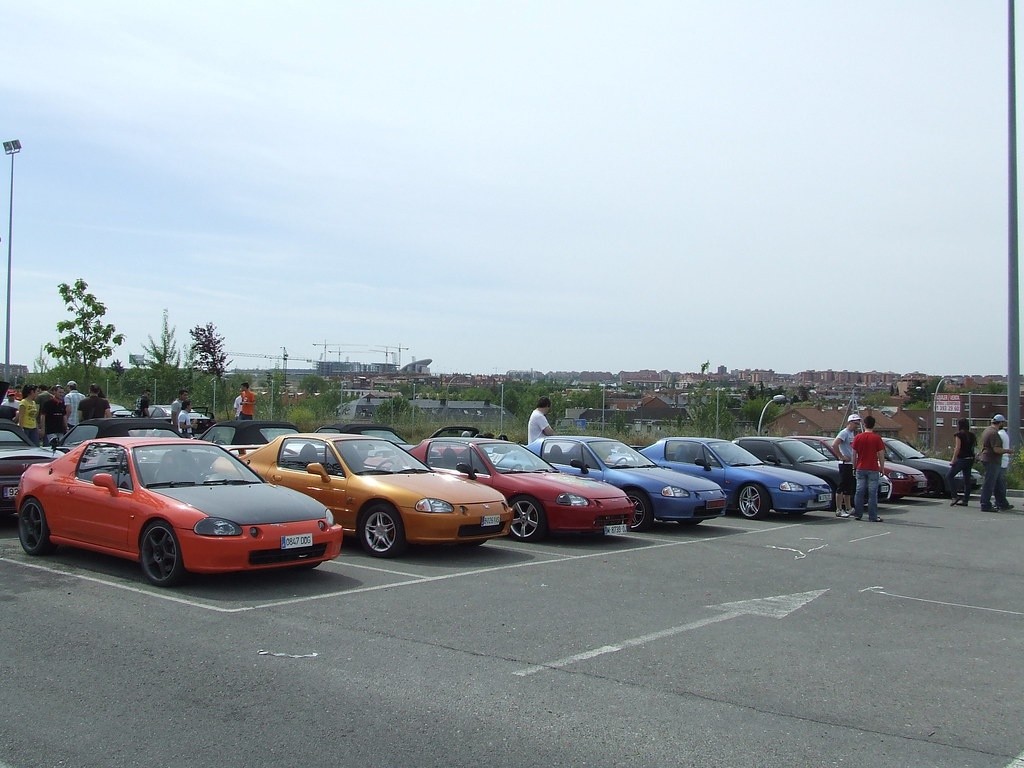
956, 502, 968, 506
992, 503, 1015, 511
950, 496, 960, 506
869, 517, 883, 522
981, 507, 999, 512
855, 516, 861, 520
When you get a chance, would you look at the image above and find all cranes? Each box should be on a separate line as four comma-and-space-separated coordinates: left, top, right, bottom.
219, 346, 313, 392
312, 338, 410, 374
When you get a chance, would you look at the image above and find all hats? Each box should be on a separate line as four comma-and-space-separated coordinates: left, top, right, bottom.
7, 389, 15, 396
66, 381, 77, 388
991, 414, 1006, 423
848, 414, 861, 423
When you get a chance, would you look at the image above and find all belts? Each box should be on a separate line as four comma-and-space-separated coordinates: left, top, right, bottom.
963, 457, 973, 459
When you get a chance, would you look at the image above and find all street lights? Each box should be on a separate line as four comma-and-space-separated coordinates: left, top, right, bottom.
932, 376, 959, 453
598, 384, 605, 435
756, 394, 786, 437
3, 138, 23, 383
714, 387, 726, 439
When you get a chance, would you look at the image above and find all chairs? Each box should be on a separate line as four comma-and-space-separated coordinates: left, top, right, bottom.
550, 445, 601, 469
444, 448, 492, 475
298, 444, 364, 475
674, 445, 696, 463
154, 450, 204, 483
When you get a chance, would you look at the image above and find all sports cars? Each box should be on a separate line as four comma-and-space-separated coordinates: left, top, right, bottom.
211, 431, 516, 559
364, 437, 636, 543
606, 434, 833, 520
13, 437, 343, 590
488, 433, 729, 534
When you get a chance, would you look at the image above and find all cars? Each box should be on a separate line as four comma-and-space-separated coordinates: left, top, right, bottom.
0, 402, 508, 513
882, 436, 984, 498
711, 433, 893, 507
784, 434, 929, 501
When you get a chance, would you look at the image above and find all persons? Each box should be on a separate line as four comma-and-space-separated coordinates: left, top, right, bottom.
946, 419, 977, 507
0, 381, 112, 446
171, 390, 198, 435
528, 397, 554, 445
234, 383, 255, 420
979, 415, 1014, 512
832, 414, 885, 522
136, 390, 151, 418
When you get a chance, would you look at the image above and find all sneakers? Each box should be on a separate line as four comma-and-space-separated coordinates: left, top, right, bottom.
846, 508, 856, 514
836, 509, 850, 517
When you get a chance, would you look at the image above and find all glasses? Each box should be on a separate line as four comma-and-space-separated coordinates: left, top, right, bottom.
851, 421, 860, 425
56, 385, 63, 388
